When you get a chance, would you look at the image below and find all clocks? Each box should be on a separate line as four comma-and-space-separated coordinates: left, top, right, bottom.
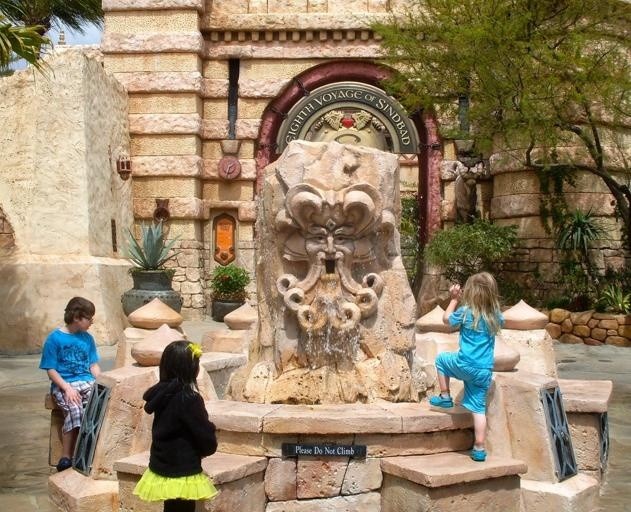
218, 156, 241, 180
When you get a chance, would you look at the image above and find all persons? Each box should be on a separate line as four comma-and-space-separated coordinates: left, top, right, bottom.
429, 270, 507, 462
38, 296, 103, 471
133, 339, 220, 511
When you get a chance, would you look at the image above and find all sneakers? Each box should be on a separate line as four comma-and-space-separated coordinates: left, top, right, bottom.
429, 395, 453, 408
470, 450, 487, 461
57, 457, 72, 472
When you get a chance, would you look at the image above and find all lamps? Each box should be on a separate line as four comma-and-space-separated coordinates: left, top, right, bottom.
108, 144, 132, 181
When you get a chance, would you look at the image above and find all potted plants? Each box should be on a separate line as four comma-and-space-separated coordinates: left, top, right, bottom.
209, 263, 251, 322
121, 218, 185, 316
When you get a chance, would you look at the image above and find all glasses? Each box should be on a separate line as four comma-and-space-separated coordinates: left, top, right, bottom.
82, 315, 93, 322
